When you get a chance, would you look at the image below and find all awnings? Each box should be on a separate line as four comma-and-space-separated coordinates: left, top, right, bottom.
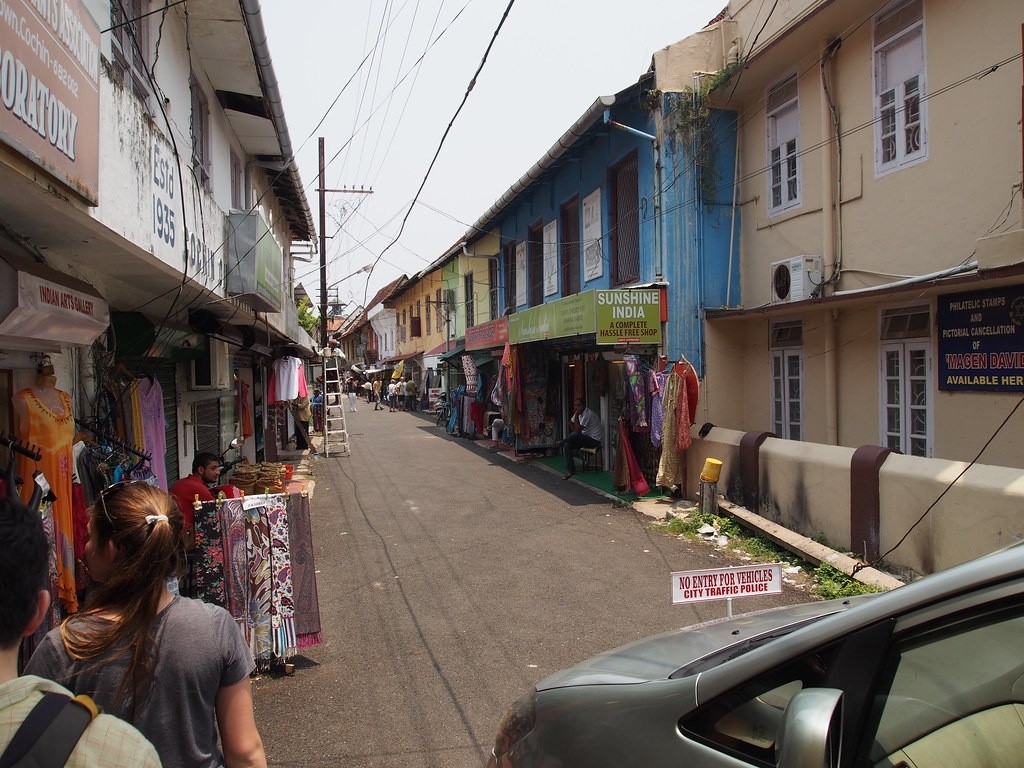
383, 350, 425, 370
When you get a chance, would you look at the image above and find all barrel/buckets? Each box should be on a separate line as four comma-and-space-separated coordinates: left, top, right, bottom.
700, 458, 722, 483
282, 465, 295, 480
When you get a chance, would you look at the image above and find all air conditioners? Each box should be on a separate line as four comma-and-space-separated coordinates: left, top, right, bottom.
191, 333, 230, 389
770, 255, 821, 304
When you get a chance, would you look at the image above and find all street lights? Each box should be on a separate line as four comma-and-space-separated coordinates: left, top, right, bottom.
320, 263, 375, 415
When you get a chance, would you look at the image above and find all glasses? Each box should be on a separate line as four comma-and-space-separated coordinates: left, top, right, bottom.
96, 479, 134, 530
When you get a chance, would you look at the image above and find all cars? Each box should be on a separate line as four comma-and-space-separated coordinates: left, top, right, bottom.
483, 536, 1024, 768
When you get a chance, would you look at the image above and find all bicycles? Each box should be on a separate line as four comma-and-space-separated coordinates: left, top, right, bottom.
436, 400, 451, 427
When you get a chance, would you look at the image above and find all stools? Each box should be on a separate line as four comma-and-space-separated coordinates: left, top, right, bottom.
581, 446, 603, 473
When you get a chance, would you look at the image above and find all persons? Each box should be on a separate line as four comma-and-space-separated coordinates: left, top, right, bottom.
24, 482, 268, 768
291, 390, 311, 450
550, 397, 602, 480
345, 375, 417, 412
15, 370, 75, 613
0, 494, 164, 767
483, 419, 507, 452
168, 453, 222, 546
310, 339, 347, 432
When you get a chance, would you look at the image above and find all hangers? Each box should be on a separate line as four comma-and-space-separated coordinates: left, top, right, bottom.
84, 426, 144, 471
284, 353, 289, 361
0, 440, 57, 511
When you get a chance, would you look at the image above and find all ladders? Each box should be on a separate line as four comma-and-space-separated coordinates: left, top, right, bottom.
322, 356, 351, 459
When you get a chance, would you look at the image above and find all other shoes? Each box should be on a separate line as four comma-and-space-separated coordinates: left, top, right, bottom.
380, 407, 384, 410
393, 410, 397, 412
354, 410, 357, 412
375, 409, 378, 410
390, 410, 392, 412
563, 471, 573, 480
